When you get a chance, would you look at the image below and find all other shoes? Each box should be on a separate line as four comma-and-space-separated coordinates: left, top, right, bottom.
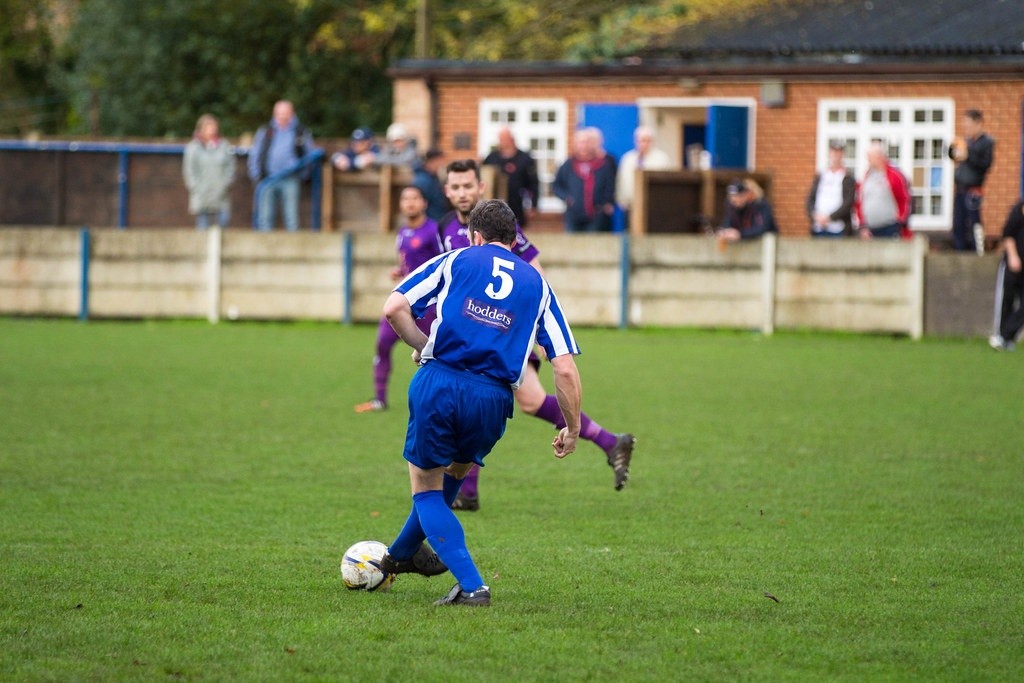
990, 335, 1005, 349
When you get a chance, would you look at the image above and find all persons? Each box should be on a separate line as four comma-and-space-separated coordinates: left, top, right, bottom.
714, 179, 780, 241
613, 126, 675, 208
551, 126, 617, 232
988, 200, 1024, 350
331, 125, 382, 171
805, 141, 856, 240
354, 187, 439, 411
853, 143, 913, 240
431, 157, 636, 511
413, 149, 447, 219
480, 125, 540, 228
949, 109, 994, 252
686, 144, 710, 171
182, 112, 238, 228
247, 100, 317, 230
387, 198, 584, 606
362, 124, 419, 167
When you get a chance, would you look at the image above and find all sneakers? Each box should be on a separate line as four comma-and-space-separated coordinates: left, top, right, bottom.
608, 432, 635, 489
431, 582, 491, 608
450, 492, 480, 511
356, 400, 387, 412
378, 546, 449, 575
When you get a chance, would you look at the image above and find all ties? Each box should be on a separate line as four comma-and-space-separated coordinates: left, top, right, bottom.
637, 153, 643, 170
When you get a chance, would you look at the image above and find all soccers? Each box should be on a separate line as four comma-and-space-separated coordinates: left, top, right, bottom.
341, 540, 395, 592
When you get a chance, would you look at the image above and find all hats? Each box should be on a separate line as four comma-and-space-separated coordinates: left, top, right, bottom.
351, 126, 371, 140
385, 123, 411, 141
